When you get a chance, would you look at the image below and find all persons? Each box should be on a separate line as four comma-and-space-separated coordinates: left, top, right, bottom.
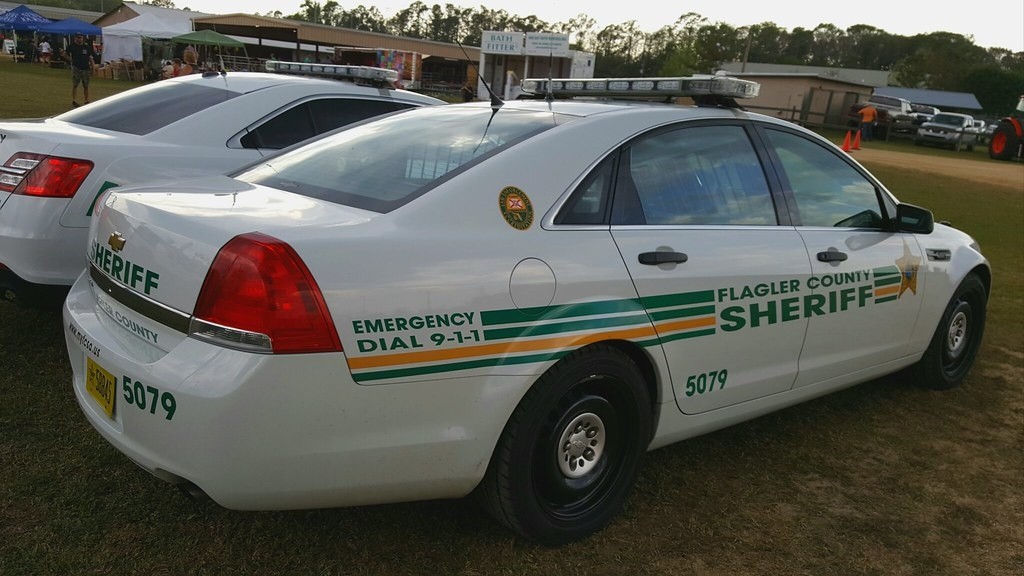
858, 102, 878, 141
63, 32, 96, 107
270, 53, 276, 60
0, 30, 11, 49
304, 55, 311, 62
28, 36, 58, 64
323, 55, 343, 64
161, 47, 200, 79
462, 82, 474, 102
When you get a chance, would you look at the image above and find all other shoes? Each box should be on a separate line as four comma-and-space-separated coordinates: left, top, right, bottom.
84, 101, 89, 104
72, 101, 78, 107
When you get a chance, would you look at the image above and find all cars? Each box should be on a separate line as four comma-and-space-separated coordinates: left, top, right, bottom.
62, 72, 993, 548
0, 60, 453, 302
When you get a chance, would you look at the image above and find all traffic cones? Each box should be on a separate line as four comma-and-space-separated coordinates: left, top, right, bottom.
851, 129, 861, 150
839, 130, 853, 153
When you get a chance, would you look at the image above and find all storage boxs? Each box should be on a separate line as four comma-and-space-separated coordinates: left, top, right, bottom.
92, 61, 132, 80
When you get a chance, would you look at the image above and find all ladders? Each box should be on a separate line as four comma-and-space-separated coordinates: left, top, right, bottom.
123, 55, 136, 82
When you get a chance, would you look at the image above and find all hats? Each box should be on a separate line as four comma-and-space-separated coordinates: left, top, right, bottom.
172, 58, 182, 63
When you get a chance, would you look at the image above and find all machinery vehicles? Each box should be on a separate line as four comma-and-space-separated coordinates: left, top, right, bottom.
988, 95, 1024, 161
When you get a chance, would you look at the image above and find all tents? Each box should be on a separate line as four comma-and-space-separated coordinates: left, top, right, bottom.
101, 12, 190, 66
0, 5, 50, 62
33, 16, 102, 69
172, 30, 250, 68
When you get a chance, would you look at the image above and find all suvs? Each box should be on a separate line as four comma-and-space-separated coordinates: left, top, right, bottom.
847, 94, 1002, 153
